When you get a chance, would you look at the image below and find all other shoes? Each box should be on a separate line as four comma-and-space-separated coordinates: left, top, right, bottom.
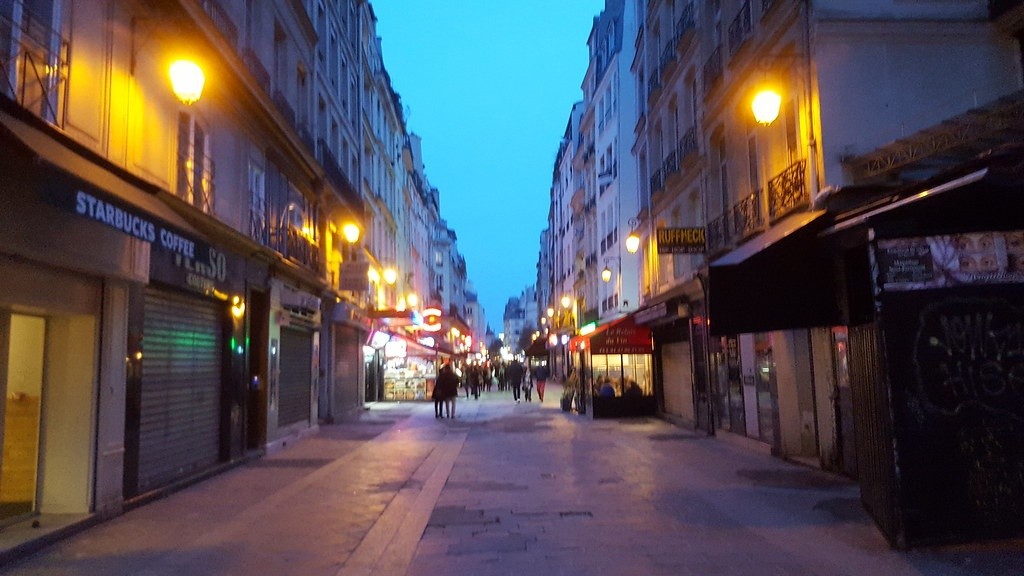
447, 414, 455, 419
514, 399, 520, 404
435, 415, 444, 419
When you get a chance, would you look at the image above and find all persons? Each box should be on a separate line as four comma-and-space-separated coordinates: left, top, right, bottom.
522, 363, 534, 402
432, 363, 460, 419
593, 374, 643, 397
461, 363, 511, 399
532, 361, 547, 403
506, 360, 523, 404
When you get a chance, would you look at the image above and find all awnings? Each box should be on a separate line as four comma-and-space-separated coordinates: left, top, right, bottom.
0, 111, 212, 267
395, 333, 453, 376
569, 316, 652, 355
